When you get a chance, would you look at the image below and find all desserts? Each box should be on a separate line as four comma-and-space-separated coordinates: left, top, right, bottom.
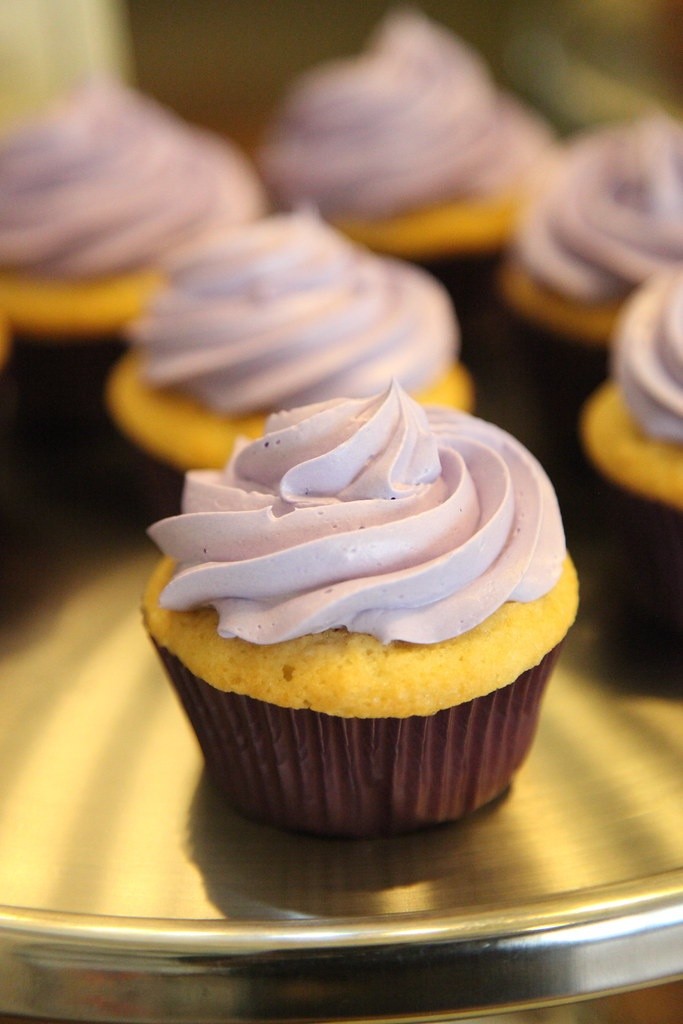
0, 10, 683, 840
